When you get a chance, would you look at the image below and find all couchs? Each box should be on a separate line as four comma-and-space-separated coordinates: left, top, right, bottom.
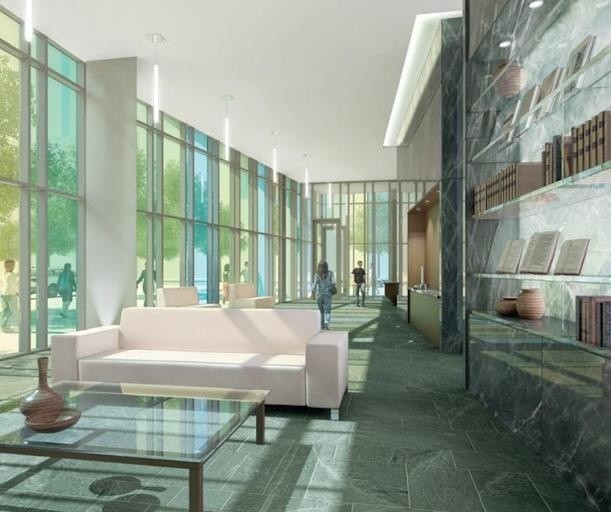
50, 306, 349, 414
228, 283, 274, 308
156, 287, 222, 307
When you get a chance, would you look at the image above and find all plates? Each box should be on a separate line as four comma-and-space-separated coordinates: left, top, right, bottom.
24, 408, 80, 432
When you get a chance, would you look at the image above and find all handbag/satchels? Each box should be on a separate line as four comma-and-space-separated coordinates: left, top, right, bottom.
328, 271, 336, 294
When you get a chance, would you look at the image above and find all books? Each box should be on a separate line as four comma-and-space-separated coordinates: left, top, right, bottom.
520, 233, 559, 275
514, 84, 542, 138
473, 109, 611, 214
574, 295, 611, 348
495, 99, 516, 154
560, 33, 597, 104
553, 238, 591, 276
535, 67, 566, 121
497, 238, 525, 275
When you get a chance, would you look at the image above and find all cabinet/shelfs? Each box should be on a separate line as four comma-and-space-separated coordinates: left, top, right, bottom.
464, 0, 611, 362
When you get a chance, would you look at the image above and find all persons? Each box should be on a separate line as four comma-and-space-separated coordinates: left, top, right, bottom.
241, 261, 250, 282
307, 260, 338, 329
137, 261, 157, 307
222, 263, 232, 308
351, 260, 369, 307
57, 263, 77, 318
4, 260, 20, 333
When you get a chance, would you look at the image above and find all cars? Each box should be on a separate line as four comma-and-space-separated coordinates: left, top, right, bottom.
31, 268, 78, 297
